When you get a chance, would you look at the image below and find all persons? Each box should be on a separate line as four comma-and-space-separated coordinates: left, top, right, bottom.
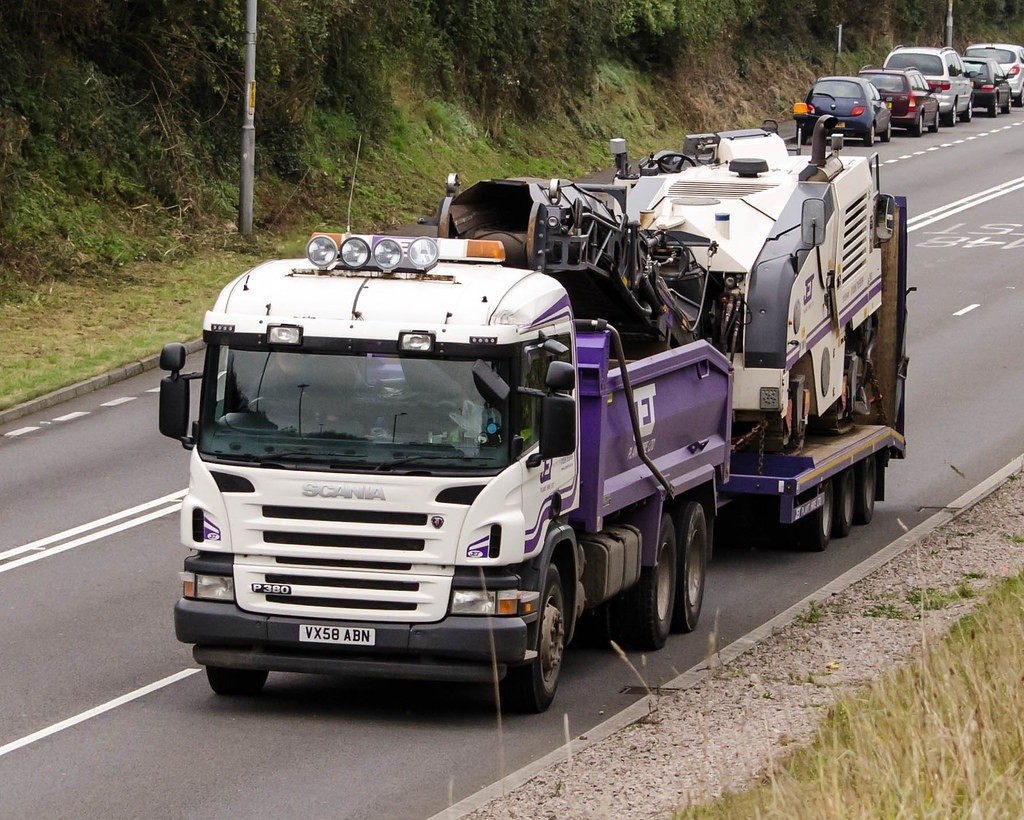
239, 352, 340, 423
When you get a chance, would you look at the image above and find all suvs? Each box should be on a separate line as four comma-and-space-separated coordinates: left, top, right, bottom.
883, 45, 976, 128
857, 65, 941, 134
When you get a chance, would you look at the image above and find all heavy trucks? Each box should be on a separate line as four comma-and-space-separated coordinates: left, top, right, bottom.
157, 112, 920, 714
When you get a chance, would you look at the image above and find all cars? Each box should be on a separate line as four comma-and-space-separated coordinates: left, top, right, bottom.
961, 44, 1024, 108
795, 76, 894, 148
960, 56, 1015, 117
